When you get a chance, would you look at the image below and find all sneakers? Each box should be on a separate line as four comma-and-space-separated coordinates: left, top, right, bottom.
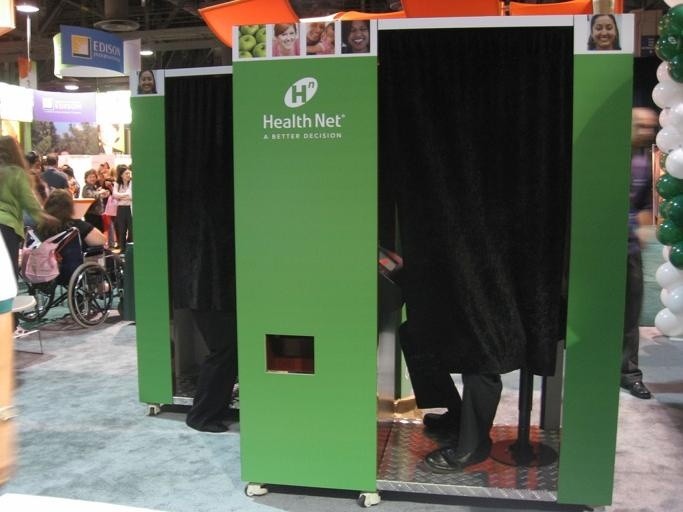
12, 325, 29, 338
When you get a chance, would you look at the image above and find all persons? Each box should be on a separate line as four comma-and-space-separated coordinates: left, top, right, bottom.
586, 14, 621, 51
272, 23, 300, 57
137, 69, 158, 94
394, 61, 558, 473
618, 105, 659, 400
184, 109, 239, 432
0, 135, 133, 328
341, 20, 370, 54
305, 22, 335, 55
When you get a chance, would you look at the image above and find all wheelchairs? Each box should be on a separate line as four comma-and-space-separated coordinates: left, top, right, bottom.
10, 224, 115, 331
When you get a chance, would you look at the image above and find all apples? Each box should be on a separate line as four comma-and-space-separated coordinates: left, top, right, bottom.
239, 25, 266, 58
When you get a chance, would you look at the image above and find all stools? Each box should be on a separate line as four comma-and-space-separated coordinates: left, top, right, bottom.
12, 293, 46, 357
485, 344, 559, 470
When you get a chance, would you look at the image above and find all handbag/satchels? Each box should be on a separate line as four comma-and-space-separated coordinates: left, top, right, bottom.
21, 244, 60, 284
104, 195, 118, 217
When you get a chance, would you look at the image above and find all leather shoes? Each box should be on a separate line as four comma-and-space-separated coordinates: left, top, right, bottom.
424, 437, 493, 472
619, 380, 650, 400
423, 409, 460, 433
